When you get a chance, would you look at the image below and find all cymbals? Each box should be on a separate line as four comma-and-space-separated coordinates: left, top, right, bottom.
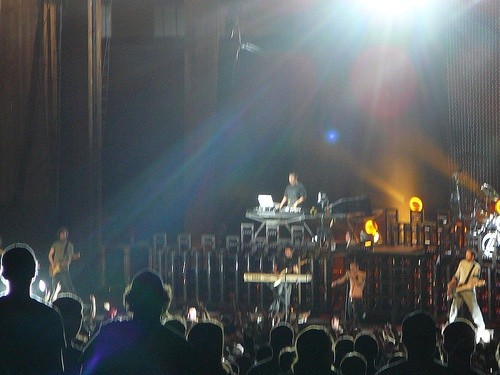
481, 184, 496, 197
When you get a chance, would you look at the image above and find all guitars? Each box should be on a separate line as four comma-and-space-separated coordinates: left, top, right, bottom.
446, 279, 485, 301
49, 251, 80, 278
273, 258, 310, 287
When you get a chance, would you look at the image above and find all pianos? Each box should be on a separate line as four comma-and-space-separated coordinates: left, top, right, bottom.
255, 205, 302, 239
243, 272, 312, 312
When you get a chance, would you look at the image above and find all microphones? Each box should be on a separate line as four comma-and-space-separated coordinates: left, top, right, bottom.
285, 268, 288, 273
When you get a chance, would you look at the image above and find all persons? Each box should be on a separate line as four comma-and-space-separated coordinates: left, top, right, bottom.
50, 223, 79, 293
0, 244, 500, 375
280, 171, 306, 209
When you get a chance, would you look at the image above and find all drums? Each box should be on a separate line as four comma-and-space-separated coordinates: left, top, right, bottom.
480, 228, 500, 258
485, 214, 500, 228
